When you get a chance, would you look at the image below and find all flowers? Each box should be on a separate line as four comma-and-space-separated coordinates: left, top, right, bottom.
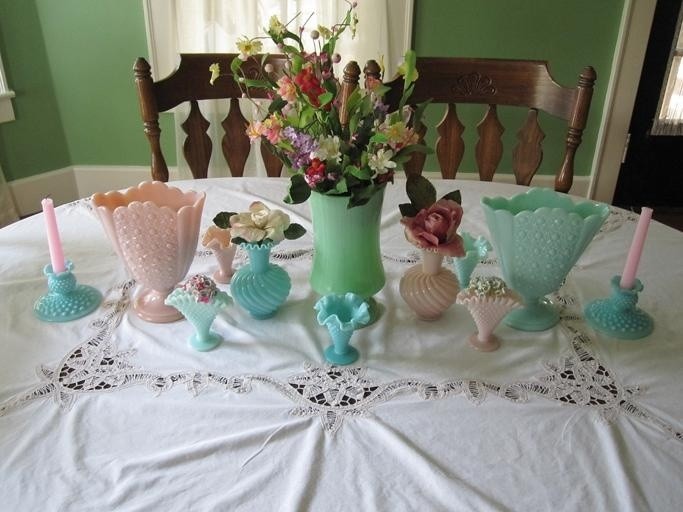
209, 2, 434, 207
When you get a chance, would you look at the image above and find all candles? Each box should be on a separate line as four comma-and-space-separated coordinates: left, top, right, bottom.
618, 203, 652, 294
41, 197, 67, 279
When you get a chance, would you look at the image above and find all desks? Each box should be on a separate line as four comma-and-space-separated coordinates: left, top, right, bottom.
0, 177, 683, 512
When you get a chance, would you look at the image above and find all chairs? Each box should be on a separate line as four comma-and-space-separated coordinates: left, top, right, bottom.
365, 58, 596, 188
132, 56, 361, 189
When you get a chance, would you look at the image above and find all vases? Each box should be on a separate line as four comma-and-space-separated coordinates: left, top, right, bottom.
307, 189, 387, 297
